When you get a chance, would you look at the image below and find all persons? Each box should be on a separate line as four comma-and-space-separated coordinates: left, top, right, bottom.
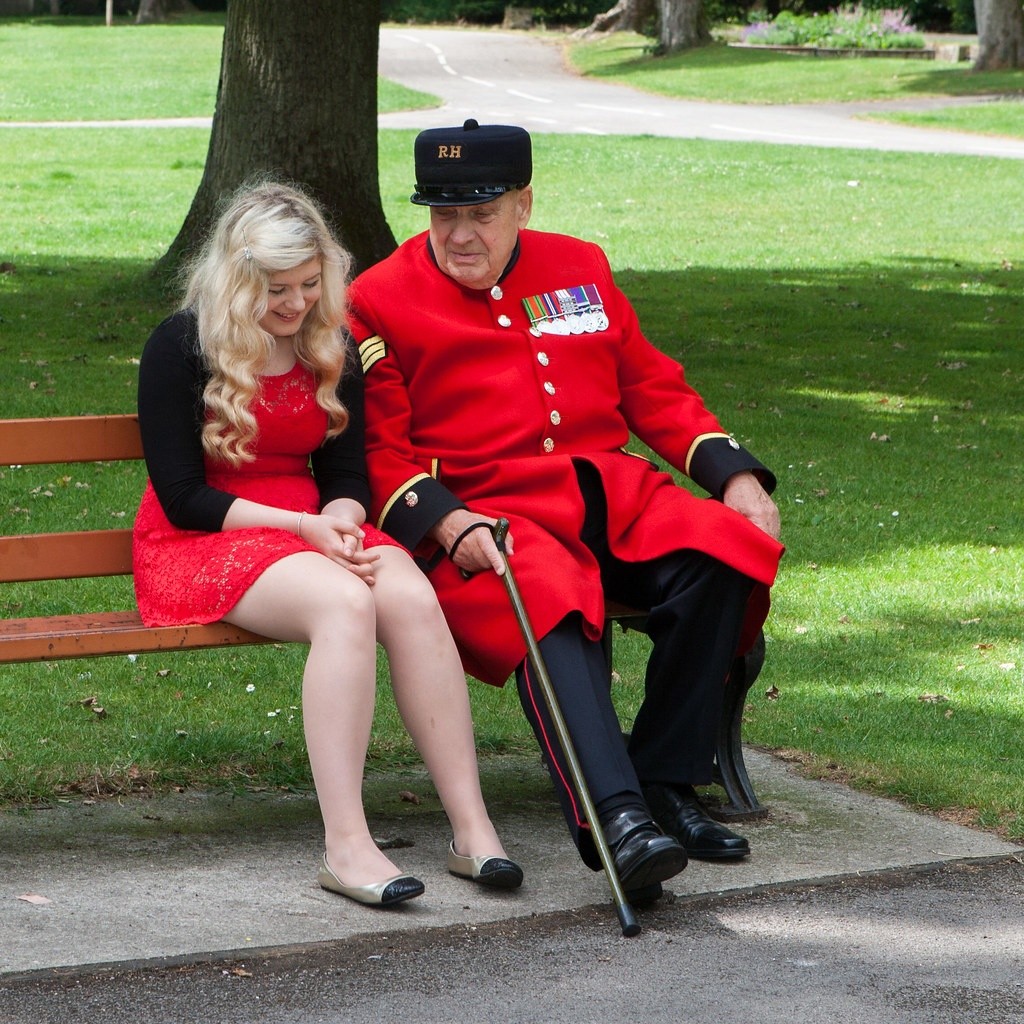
133, 181, 524, 904
345, 119, 787, 900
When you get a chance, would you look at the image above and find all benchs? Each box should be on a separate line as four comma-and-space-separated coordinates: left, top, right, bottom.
1, 413, 768, 824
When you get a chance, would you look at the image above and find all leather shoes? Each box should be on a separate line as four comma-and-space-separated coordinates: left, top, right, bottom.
597, 808, 689, 905
641, 780, 752, 857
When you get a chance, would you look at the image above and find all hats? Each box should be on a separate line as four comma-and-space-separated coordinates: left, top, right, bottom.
410, 119, 533, 207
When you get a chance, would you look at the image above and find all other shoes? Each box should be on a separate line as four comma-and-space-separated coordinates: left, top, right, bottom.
447, 838, 524, 886
318, 850, 426, 905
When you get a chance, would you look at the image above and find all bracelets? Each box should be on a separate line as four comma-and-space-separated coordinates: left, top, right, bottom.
298, 511, 307, 536
